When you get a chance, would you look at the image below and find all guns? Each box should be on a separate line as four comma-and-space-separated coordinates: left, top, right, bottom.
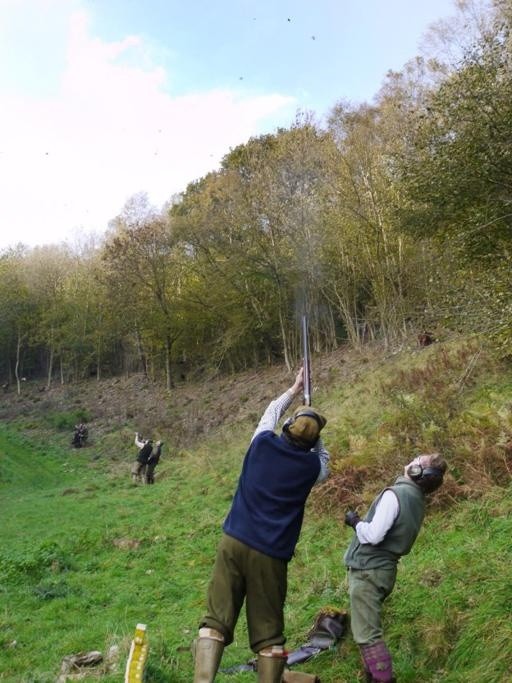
302, 315, 311, 407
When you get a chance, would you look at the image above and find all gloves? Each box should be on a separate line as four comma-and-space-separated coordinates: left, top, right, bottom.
343, 505, 363, 532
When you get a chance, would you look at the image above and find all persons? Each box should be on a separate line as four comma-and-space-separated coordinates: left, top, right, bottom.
130, 431, 153, 483
343, 454, 448, 682
193, 367, 329, 682
146, 439, 164, 483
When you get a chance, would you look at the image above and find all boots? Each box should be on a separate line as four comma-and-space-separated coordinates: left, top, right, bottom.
193, 626, 225, 683
360, 642, 392, 682
257, 654, 289, 683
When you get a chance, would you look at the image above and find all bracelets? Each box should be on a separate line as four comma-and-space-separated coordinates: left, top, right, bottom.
288, 387, 297, 396
